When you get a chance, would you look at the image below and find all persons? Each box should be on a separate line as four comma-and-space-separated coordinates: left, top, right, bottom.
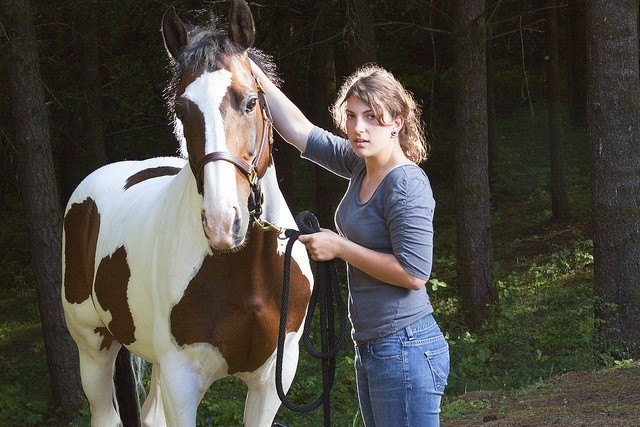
247, 56, 450, 426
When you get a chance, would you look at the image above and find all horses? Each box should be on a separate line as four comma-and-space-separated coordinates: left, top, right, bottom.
61, 0, 315, 427
